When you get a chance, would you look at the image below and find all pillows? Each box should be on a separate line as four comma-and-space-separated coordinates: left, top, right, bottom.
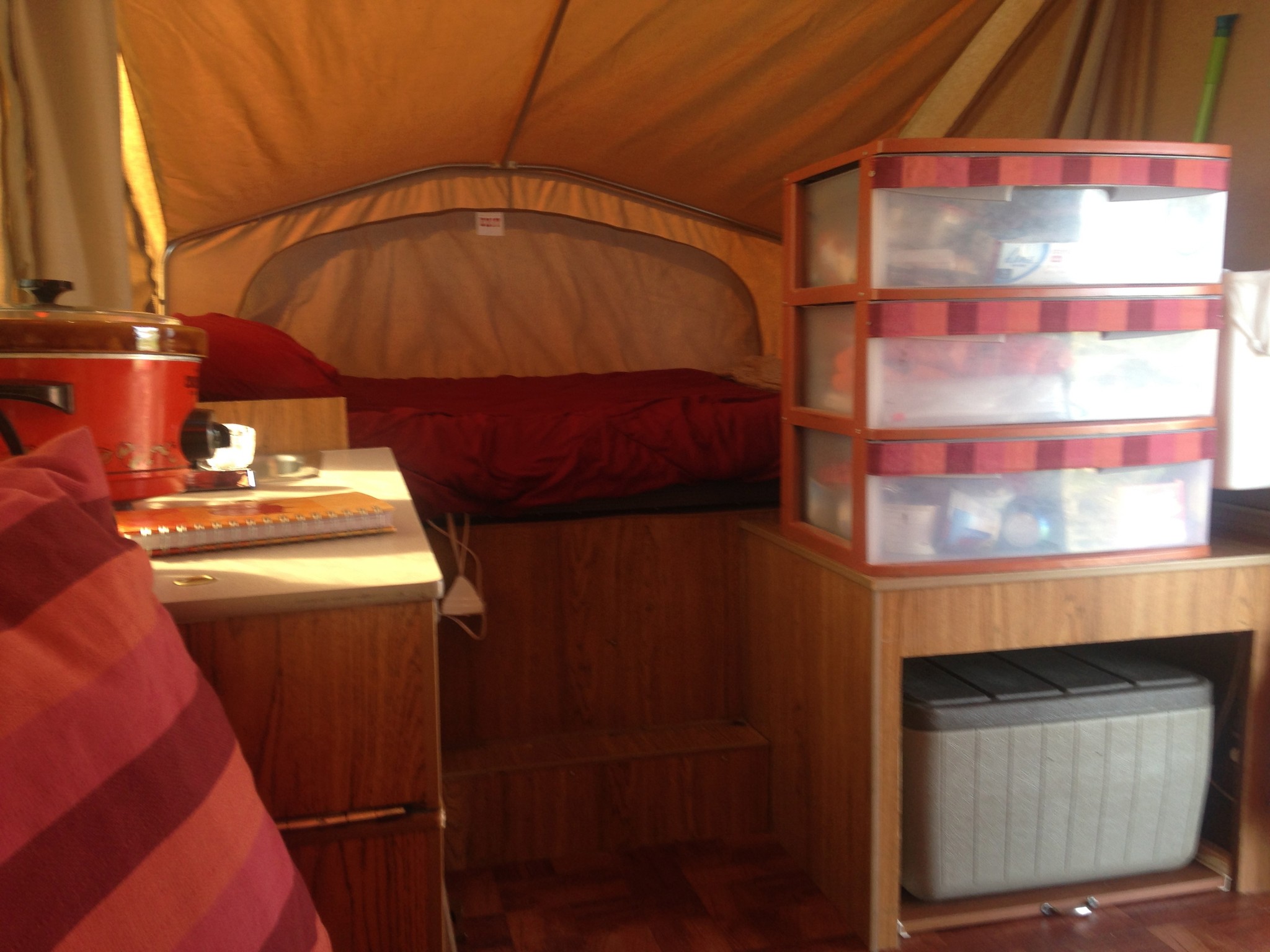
168, 307, 351, 409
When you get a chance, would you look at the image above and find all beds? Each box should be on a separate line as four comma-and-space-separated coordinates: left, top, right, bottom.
319, 363, 784, 517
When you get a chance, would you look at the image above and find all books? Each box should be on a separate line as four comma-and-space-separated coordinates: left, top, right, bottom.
115, 490, 399, 554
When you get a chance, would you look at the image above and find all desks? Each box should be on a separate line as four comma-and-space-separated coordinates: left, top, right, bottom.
139, 436, 444, 952
739, 522, 1270, 952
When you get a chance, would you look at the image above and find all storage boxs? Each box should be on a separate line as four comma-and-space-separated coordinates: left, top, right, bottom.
772, 121, 1227, 562
896, 649, 1216, 897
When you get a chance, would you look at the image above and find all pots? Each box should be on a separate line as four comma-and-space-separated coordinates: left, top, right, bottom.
1, 278, 231, 512
